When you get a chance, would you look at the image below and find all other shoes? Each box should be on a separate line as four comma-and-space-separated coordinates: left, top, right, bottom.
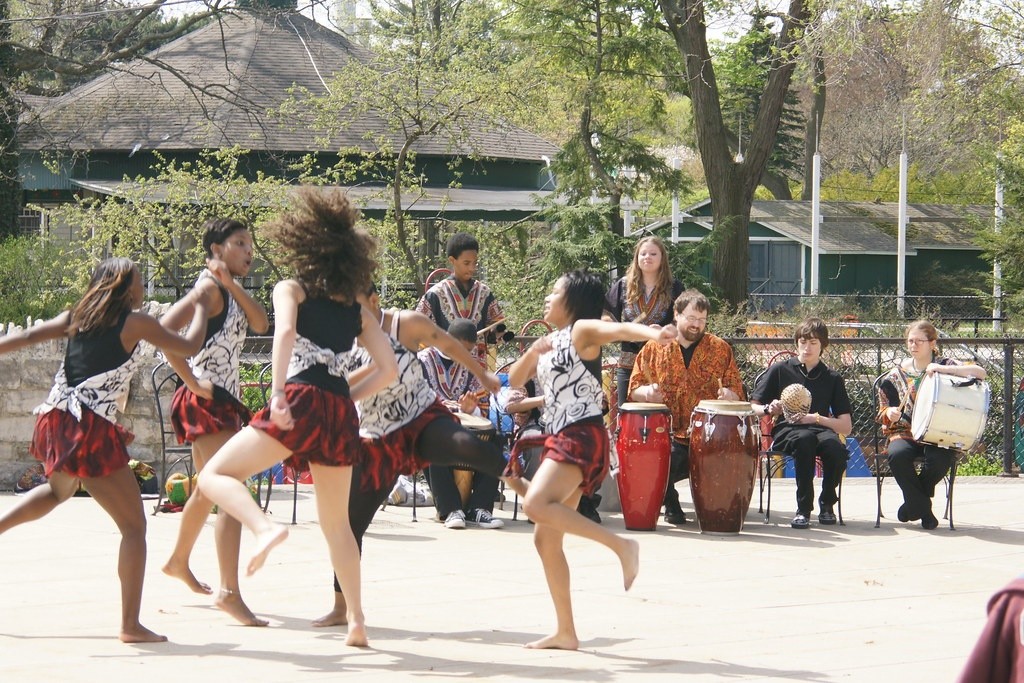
819, 498, 836, 524
790, 513, 810, 528
579, 495, 602, 523
898, 502, 909, 523
665, 508, 687, 524
920, 511, 938, 530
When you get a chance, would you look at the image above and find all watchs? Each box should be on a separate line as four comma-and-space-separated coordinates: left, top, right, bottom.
763, 403, 770, 414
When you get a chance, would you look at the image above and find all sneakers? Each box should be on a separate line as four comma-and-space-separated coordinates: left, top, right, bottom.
464, 506, 505, 528
444, 510, 464, 528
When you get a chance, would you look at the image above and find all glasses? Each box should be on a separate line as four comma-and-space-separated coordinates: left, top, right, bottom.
906, 339, 932, 345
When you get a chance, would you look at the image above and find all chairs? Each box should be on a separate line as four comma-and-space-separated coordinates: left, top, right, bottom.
493, 359, 533, 522
752, 350, 846, 527
151, 360, 218, 516
871, 369, 958, 531
259, 362, 302, 527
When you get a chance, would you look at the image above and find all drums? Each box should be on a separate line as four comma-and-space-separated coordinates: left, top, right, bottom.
451, 412, 497, 509
911, 371, 990, 454
688, 399, 759, 537
616, 401, 671, 531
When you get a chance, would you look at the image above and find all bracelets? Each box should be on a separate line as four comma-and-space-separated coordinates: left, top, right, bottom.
814, 412, 819, 425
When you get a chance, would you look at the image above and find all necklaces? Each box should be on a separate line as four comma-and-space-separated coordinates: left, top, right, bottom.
380, 308, 385, 328
798, 366, 821, 380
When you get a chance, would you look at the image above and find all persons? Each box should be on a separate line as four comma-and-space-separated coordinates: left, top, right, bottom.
415, 317, 505, 529
195, 185, 399, 649
876, 320, 988, 530
626, 287, 748, 525
509, 270, 678, 651
418, 233, 507, 376
156, 215, 271, 625
749, 317, 853, 530
313, 280, 531, 624
599, 234, 686, 404
0, 257, 213, 643
